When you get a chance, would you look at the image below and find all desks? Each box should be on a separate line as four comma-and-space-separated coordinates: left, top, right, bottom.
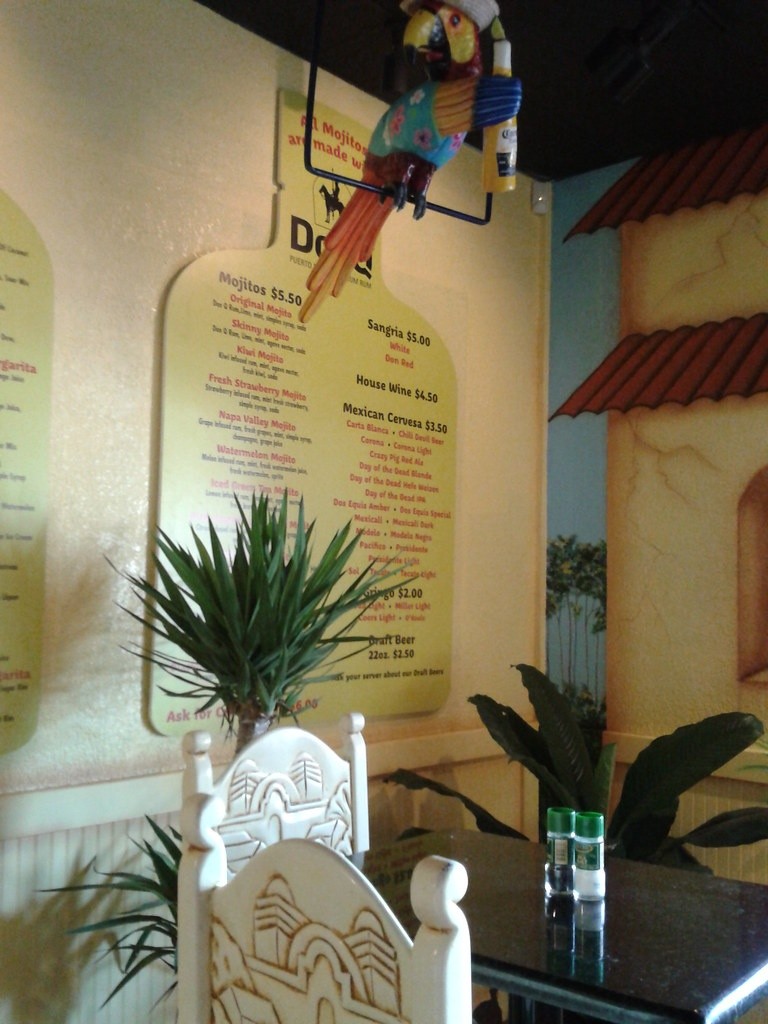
347, 829, 768, 1024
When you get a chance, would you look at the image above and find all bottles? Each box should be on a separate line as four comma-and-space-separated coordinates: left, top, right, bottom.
573, 811, 607, 900
543, 807, 576, 898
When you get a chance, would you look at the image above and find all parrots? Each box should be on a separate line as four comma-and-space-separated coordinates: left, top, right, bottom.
295, 1, 524, 324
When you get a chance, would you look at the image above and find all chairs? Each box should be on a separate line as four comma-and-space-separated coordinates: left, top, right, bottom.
176, 710, 473, 1024
